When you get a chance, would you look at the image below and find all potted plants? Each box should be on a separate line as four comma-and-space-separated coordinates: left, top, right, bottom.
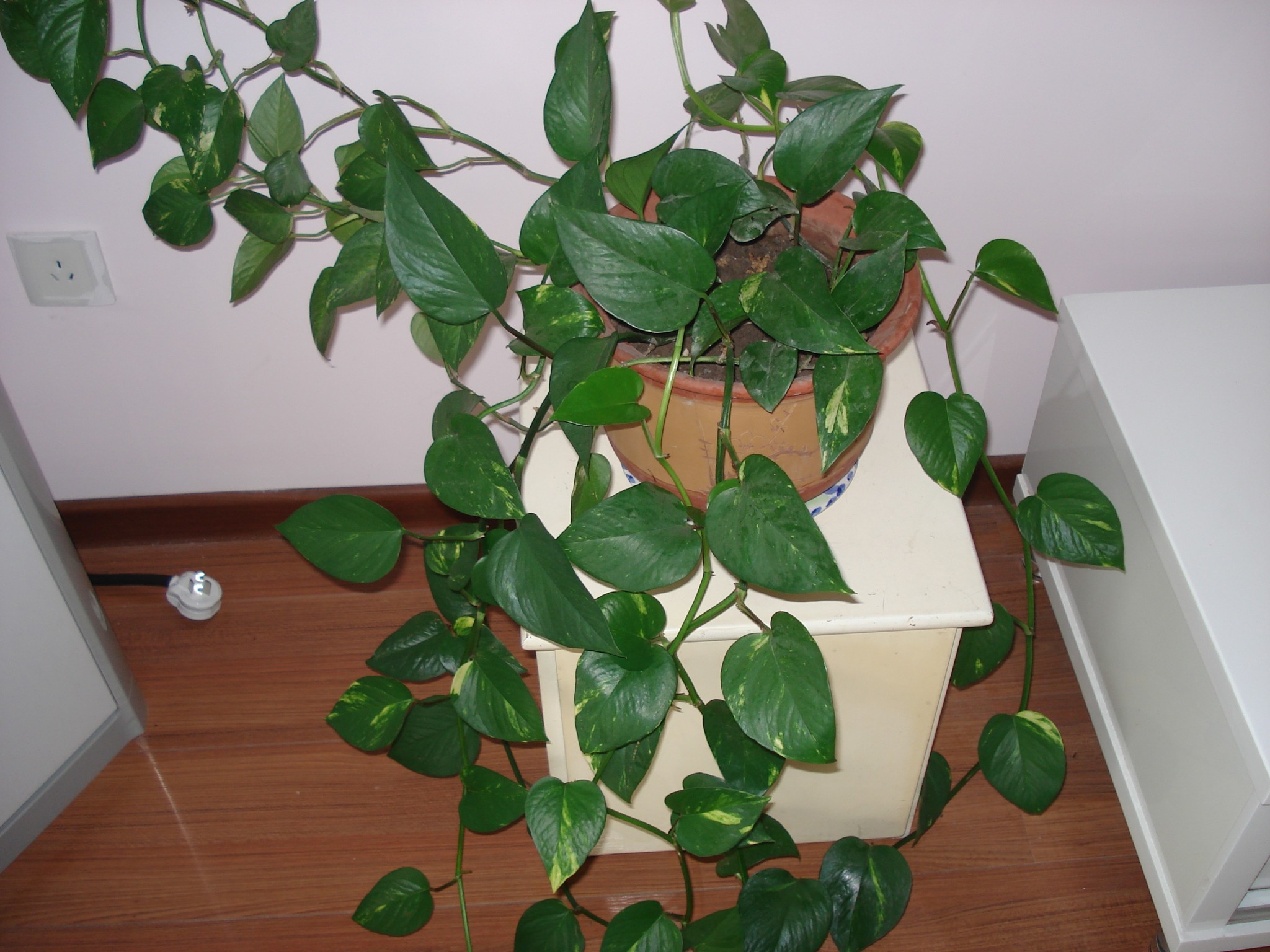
0, 0, 1123, 952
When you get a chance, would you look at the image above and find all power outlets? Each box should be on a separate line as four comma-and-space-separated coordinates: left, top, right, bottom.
6, 231, 115, 306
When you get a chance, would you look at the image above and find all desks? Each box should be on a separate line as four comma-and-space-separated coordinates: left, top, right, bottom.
520, 329, 994, 855
